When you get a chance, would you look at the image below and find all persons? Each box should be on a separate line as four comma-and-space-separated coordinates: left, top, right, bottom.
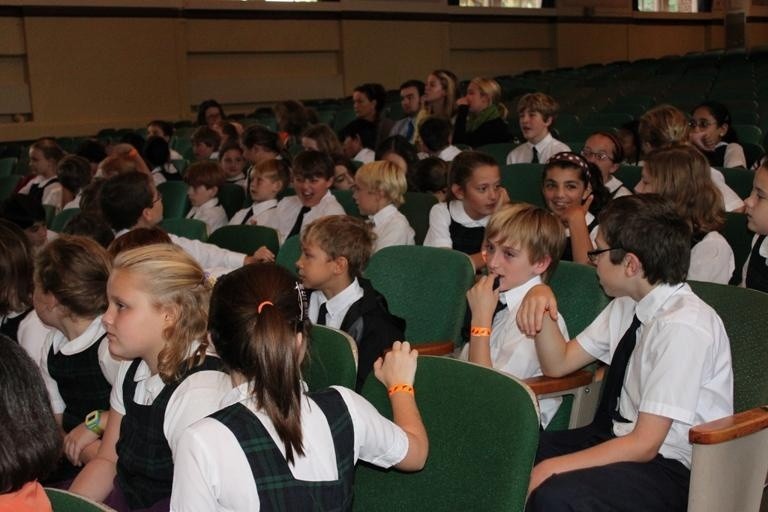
163, 259, 430, 512
65, 243, 231, 511
0, 334, 67, 510
0, 66, 767, 468
517, 194, 734, 512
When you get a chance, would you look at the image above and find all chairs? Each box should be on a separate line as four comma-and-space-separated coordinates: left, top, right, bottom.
0, 44, 768, 512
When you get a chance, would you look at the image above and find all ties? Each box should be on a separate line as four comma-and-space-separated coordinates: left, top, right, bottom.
594, 313, 640, 423
240, 207, 254, 226
316, 302, 327, 325
190, 212, 195, 219
405, 120, 414, 141
460, 275, 506, 343
287, 205, 310, 238
531, 147, 538, 164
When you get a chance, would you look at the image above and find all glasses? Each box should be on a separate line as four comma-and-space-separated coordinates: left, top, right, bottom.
580, 149, 613, 162
206, 113, 220, 121
586, 246, 620, 262
687, 120, 718, 128
151, 191, 162, 204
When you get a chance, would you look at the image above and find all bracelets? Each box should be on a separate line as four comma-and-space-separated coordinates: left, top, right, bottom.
386, 382, 416, 398
